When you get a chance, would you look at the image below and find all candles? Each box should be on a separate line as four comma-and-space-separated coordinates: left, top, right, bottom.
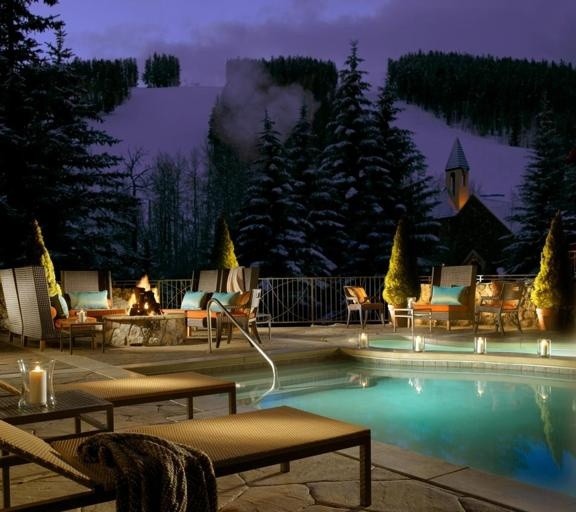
360, 333, 367, 348
539, 340, 549, 356
477, 337, 484, 353
416, 335, 422, 352
27, 365, 48, 406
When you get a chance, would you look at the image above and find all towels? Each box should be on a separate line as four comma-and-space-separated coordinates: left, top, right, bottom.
73, 428, 219, 512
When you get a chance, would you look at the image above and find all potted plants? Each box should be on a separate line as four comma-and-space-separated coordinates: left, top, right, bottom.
530, 209, 569, 333
381, 216, 416, 327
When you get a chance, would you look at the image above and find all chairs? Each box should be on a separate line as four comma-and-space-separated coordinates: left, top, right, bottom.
0, 369, 238, 426
0, 264, 262, 354
0, 404, 374, 512
344, 264, 525, 337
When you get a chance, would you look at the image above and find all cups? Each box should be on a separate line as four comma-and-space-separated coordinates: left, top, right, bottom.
474, 337, 486, 354
359, 374, 370, 387
472, 381, 489, 401
537, 338, 552, 358
359, 333, 369, 349
16, 357, 57, 408
407, 297, 417, 309
538, 384, 551, 403
414, 335, 426, 352
77, 311, 87, 324
409, 377, 425, 390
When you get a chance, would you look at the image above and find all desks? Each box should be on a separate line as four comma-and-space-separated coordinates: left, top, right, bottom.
0, 389, 115, 508
